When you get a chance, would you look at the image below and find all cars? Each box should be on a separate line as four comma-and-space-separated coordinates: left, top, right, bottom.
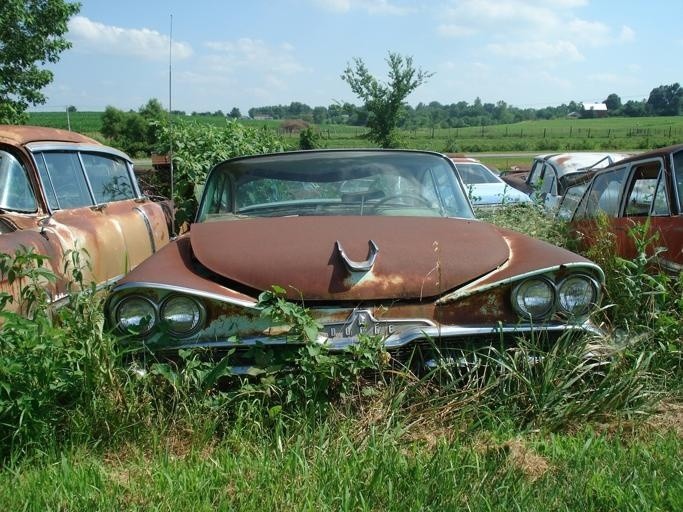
0, 125, 187, 349
562, 143, 682, 278
101, 147, 604, 385
396, 152, 673, 215
